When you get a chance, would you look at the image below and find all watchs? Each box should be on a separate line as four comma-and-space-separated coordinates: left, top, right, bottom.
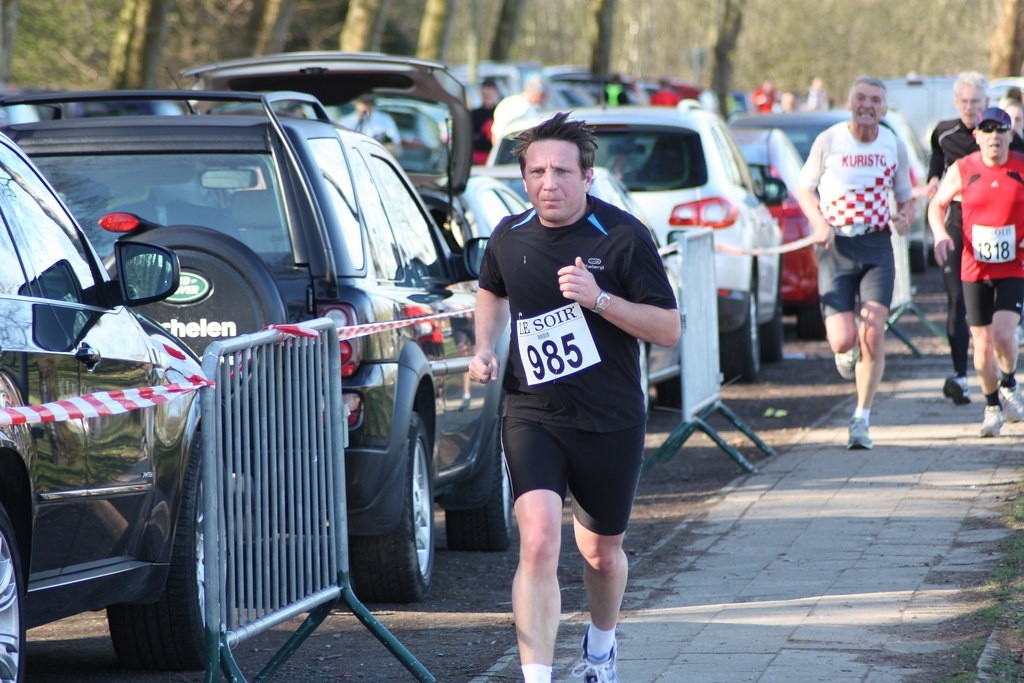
592, 290, 610, 313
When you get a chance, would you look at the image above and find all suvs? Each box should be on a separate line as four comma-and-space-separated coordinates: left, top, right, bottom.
0, 83, 518, 606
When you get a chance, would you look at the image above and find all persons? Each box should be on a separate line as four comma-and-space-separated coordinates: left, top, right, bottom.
341, 92, 403, 158
751, 77, 775, 113
651, 78, 681, 106
443, 283, 476, 412
928, 107, 1024, 438
485, 79, 550, 166
998, 97, 1024, 142
925, 71, 1024, 405
467, 111, 681, 683
471, 79, 500, 166
605, 73, 628, 107
399, 303, 447, 411
806, 77, 828, 113
797, 77, 915, 449
781, 90, 797, 113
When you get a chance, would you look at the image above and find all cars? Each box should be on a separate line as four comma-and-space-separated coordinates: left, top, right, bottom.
0, 128, 251, 683
179, 49, 1024, 621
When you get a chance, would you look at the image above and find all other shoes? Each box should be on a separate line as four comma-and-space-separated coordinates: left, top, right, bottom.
1014, 324, 1024, 345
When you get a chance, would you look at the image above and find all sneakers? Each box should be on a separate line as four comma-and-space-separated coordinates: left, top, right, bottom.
846, 415, 874, 449
834, 326, 861, 379
979, 405, 1004, 437
997, 378, 1024, 423
572, 625, 618, 683
943, 374, 970, 404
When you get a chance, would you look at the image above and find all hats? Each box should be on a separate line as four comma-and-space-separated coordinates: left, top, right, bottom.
975, 107, 1013, 132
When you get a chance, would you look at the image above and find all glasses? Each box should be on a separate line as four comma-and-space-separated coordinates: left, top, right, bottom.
978, 122, 1009, 133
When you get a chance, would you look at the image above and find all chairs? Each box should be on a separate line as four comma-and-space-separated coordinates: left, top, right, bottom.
230, 190, 289, 263
